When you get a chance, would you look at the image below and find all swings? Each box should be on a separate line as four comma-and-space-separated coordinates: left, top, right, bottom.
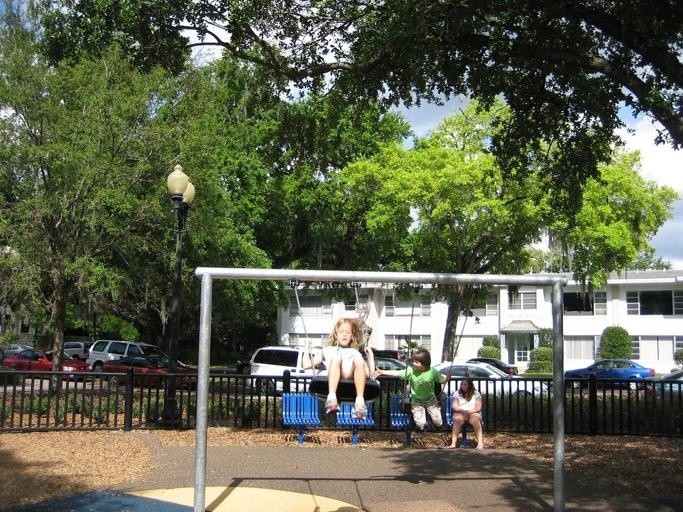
396, 281, 481, 416
288, 280, 383, 404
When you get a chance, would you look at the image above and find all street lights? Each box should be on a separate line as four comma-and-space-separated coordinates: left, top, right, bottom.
165, 162, 196, 428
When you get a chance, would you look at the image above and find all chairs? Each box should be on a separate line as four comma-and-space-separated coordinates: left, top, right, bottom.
390, 395, 429, 446
445, 395, 485, 446
283, 393, 320, 445
338, 404, 373, 447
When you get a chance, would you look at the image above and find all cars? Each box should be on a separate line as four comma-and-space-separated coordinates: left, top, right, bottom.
0, 339, 196, 392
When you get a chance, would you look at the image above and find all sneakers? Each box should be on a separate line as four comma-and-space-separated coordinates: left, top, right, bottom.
351, 406, 368, 420
432, 423, 447, 432
324, 397, 339, 414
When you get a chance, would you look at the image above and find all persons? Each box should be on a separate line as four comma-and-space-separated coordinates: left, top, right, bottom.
442, 377, 482, 450
309, 317, 374, 419
373, 350, 451, 431
